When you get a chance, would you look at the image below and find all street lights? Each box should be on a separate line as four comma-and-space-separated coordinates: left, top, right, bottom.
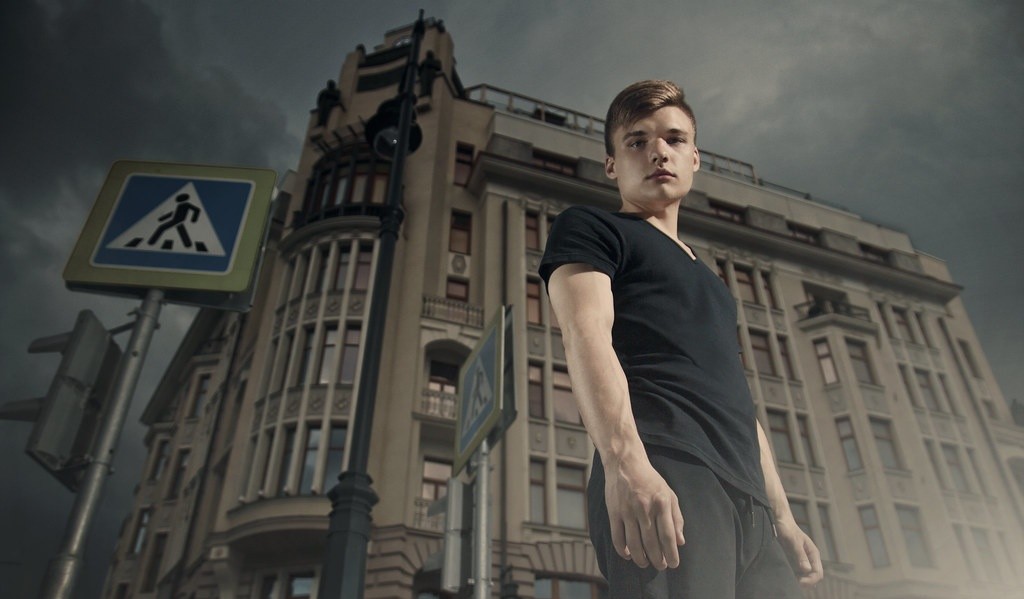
318, 10, 425, 599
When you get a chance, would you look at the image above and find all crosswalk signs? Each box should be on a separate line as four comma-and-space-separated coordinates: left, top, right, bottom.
65, 160, 278, 313
450, 307, 506, 475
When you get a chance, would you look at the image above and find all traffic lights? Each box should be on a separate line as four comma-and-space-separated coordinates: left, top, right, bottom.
0, 309, 123, 494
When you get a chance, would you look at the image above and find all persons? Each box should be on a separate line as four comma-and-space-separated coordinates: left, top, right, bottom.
317, 79, 346, 127
538, 79, 824, 599
418, 50, 443, 97
